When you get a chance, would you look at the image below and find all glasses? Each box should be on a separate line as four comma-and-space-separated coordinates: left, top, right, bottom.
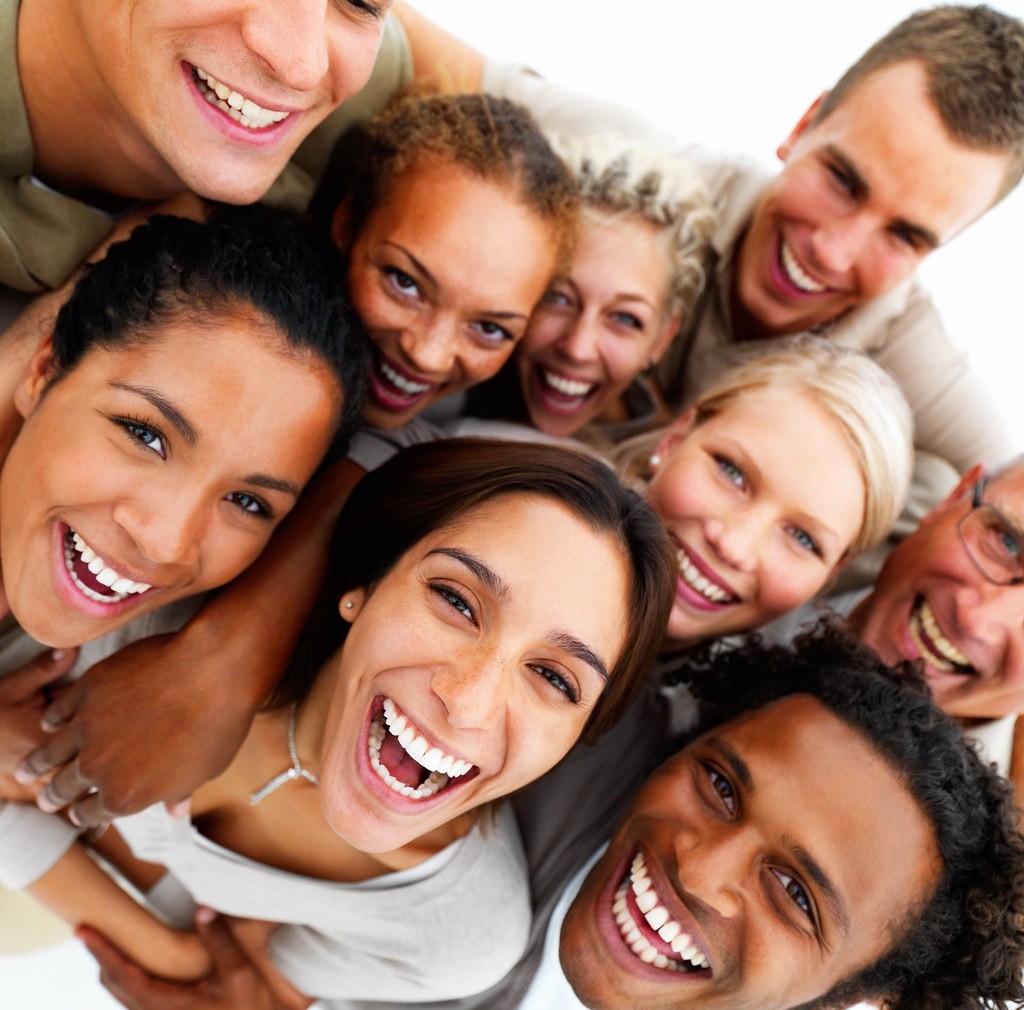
957, 471, 1024, 587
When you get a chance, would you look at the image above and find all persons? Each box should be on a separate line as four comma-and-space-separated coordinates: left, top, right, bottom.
0, 2, 1023, 833
79, 621, 1024, 1009
0, 437, 677, 1010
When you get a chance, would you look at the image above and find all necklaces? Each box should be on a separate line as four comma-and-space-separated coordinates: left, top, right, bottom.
249, 703, 320, 807
